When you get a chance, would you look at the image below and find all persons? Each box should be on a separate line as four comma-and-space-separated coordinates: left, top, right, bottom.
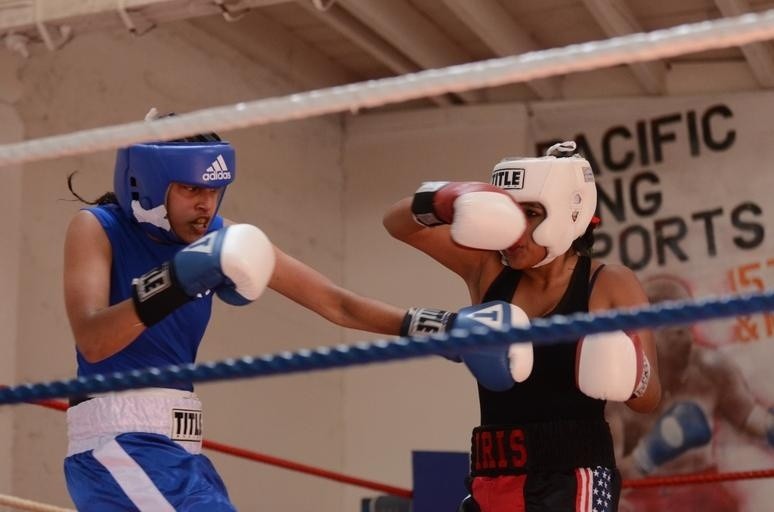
603, 276, 773, 511
62, 107, 534, 512
380, 140, 661, 512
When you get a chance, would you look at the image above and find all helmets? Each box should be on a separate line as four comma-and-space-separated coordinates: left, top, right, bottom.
113, 140, 236, 238
490, 141, 598, 267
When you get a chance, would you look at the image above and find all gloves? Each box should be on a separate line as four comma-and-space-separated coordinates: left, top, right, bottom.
630, 401, 711, 471
130, 223, 275, 328
575, 329, 650, 403
401, 301, 535, 392
412, 180, 530, 253
